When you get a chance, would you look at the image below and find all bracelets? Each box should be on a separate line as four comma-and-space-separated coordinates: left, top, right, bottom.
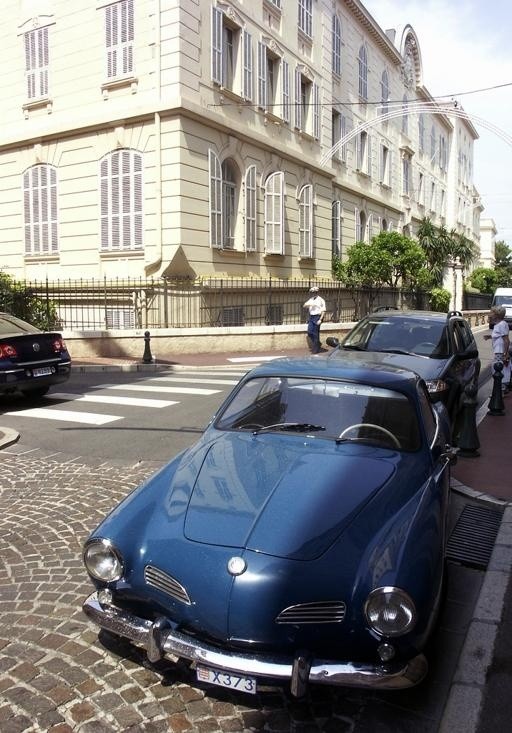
503, 352, 507, 356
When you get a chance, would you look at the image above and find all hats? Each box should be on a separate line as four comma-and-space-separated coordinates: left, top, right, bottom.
310, 287, 319, 292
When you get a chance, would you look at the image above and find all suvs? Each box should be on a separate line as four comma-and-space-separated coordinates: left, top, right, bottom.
326, 305, 481, 441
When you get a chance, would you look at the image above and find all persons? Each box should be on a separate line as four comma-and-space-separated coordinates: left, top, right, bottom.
302, 287, 326, 354
483, 305, 510, 399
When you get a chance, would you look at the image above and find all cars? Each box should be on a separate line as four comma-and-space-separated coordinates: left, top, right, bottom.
81, 354, 461, 698
0, 311, 72, 401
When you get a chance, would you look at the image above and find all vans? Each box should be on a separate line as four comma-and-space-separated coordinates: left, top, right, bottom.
488, 288, 512, 329
